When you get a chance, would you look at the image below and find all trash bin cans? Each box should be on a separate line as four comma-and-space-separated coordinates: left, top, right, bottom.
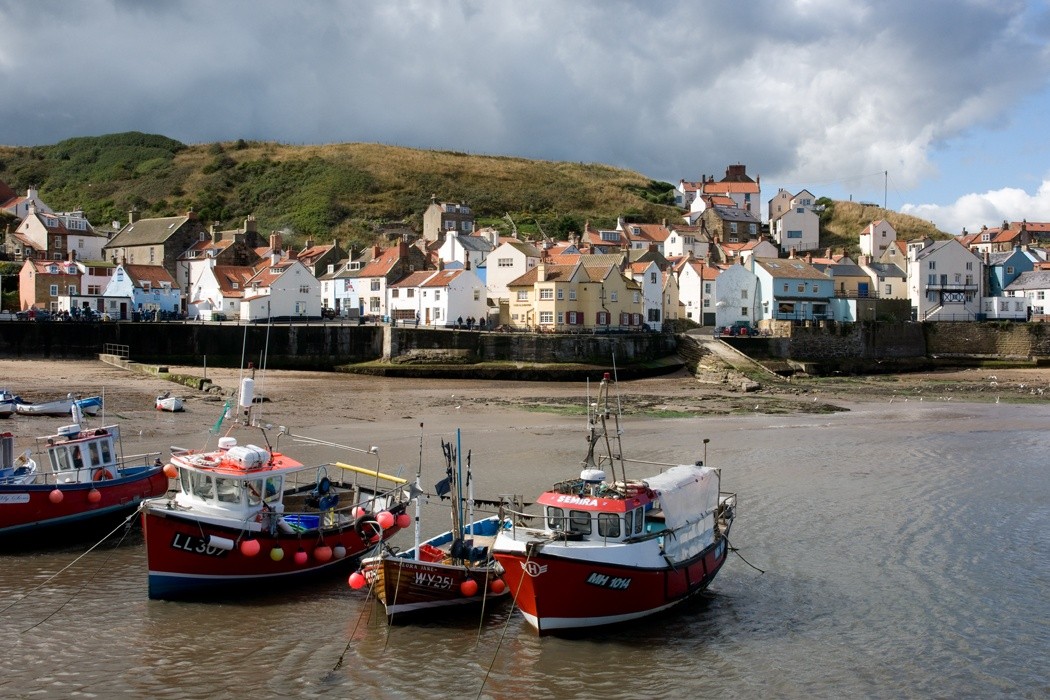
131, 312, 140, 322
213, 313, 226, 321
360, 317, 365, 324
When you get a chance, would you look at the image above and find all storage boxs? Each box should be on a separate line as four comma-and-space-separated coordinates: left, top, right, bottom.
419, 544, 444, 562
442, 557, 470, 566
284, 515, 319, 529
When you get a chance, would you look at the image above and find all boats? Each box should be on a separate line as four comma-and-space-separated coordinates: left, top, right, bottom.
492, 352, 739, 640
138, 298, 413, 602
0, 384, 172, 552
0, 388, 103, 420
359, 426, 530, 627
154, 391, 183, 412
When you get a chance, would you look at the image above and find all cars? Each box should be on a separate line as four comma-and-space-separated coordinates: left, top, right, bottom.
727, 325, 760, 336
80, 308, 111, 321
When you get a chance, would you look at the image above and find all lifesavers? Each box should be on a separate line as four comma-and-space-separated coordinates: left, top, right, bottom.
617, 486, 647, 494
196, 452, 222, 467
354, 515, 379, 539
93, 469, 113, 481
614, 479, 649, 490
